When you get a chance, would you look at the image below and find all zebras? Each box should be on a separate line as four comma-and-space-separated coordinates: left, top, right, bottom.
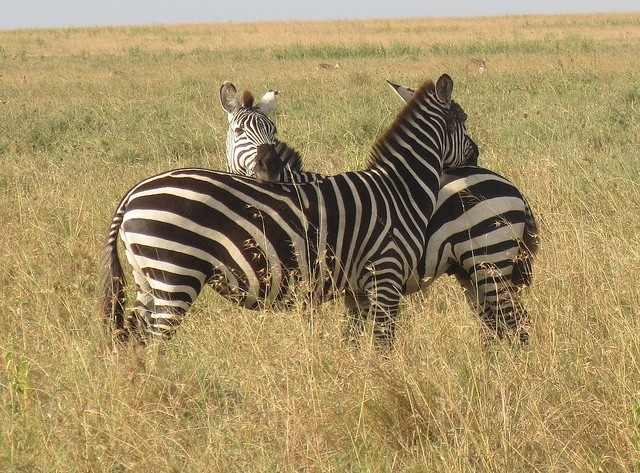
101, 74, 479, 367
219, 80, 540, 351
